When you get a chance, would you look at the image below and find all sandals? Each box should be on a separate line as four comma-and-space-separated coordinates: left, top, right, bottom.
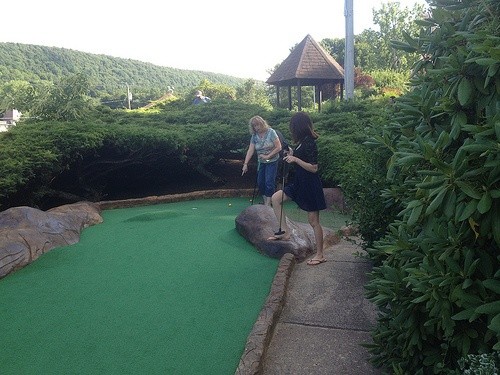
308, 256, 327, 265
268, 232, 290, 240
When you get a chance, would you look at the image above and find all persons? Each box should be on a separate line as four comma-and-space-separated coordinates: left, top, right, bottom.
268, 111, 327, 265
241, 116, 283, 209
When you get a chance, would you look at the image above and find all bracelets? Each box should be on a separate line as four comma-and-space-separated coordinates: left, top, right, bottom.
243, 163, 248, 165
